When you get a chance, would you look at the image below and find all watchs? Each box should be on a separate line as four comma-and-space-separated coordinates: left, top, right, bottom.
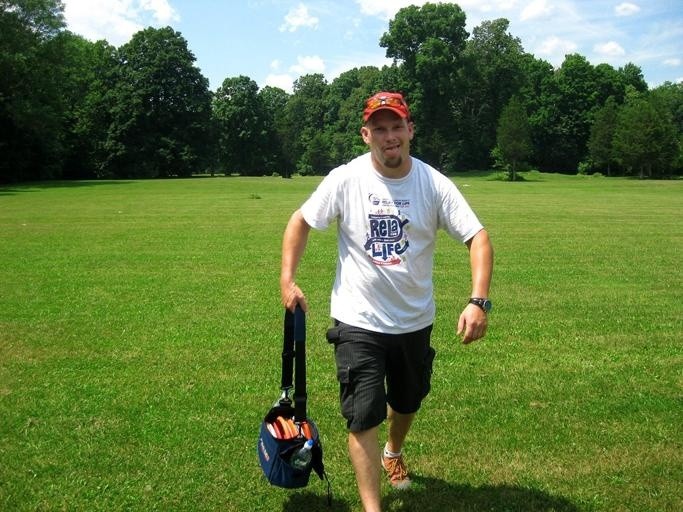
468, 297, 492, 313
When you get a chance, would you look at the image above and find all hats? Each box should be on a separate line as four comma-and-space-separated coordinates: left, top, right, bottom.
363, 90, 411, 124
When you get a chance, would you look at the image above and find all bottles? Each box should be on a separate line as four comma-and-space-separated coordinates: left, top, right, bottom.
290, 439, 314, 470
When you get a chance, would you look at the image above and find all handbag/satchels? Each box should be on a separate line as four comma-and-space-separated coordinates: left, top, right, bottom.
256, 301, 326, 489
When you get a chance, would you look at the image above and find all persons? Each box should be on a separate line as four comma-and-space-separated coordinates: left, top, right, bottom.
279, 92, 494, 512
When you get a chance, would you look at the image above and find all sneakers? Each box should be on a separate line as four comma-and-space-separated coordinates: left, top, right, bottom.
379, 449, 412, 492
